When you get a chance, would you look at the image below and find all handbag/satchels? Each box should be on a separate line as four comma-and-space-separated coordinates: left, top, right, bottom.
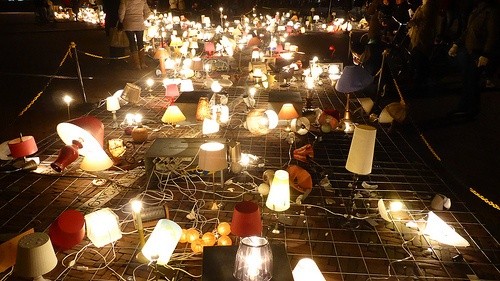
110, 26, 129, 48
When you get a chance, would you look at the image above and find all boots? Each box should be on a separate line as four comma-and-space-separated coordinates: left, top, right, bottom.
131, 50, 141, 71
139, 48, 149, 69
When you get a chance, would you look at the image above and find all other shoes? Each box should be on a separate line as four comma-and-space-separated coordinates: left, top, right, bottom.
367, 39, 376, 44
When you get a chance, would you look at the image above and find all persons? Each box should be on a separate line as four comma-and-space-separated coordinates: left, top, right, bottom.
364, 0, 500, 88
102, 0, 151, 69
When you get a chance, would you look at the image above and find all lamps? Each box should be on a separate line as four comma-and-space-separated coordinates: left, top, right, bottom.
0, 6, 471, 281
